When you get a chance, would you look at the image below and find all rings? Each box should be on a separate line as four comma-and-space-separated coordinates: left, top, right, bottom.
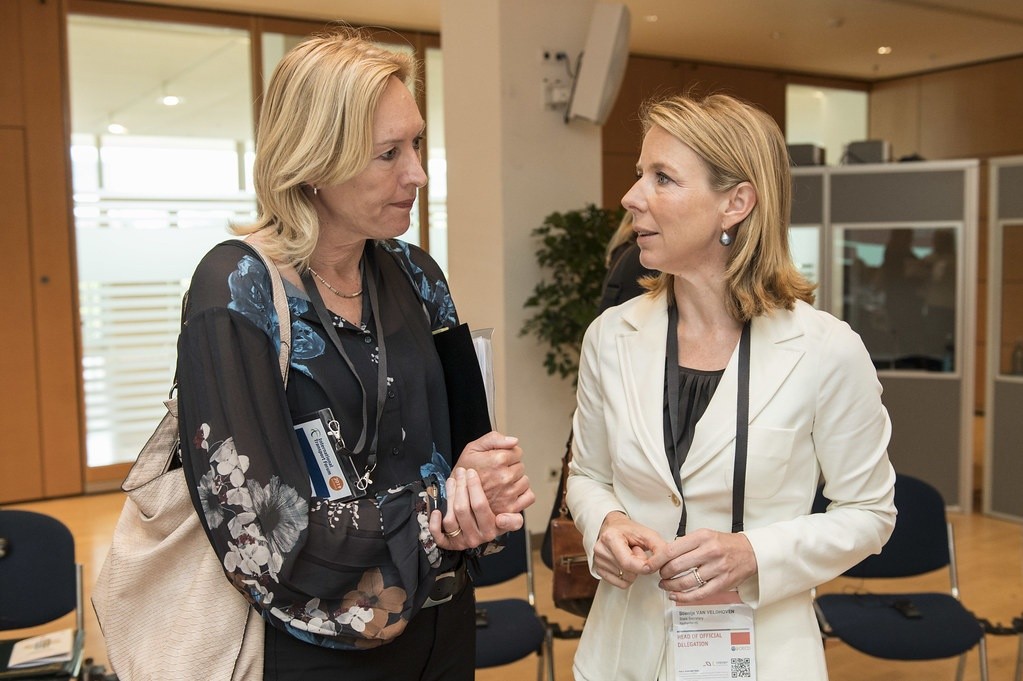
619, 570, 623, 579
445, 529, 462, 538
693, 569, 705, 586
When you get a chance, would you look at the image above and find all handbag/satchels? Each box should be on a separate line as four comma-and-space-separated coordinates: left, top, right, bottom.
555, 600, 594, 618
540, 464, 571, 570
550, 518, 601, 601
91, 239, 290, 681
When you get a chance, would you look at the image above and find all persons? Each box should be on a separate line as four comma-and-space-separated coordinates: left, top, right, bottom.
564, 91, 900, 681
177, 30, 536, 681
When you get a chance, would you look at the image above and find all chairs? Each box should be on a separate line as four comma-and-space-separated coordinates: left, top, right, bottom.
467, 508, 554, 681
810, 473, 988, 681
0, 509, 86, 681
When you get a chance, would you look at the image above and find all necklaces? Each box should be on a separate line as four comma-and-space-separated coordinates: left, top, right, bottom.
307, 266, 363, 298
